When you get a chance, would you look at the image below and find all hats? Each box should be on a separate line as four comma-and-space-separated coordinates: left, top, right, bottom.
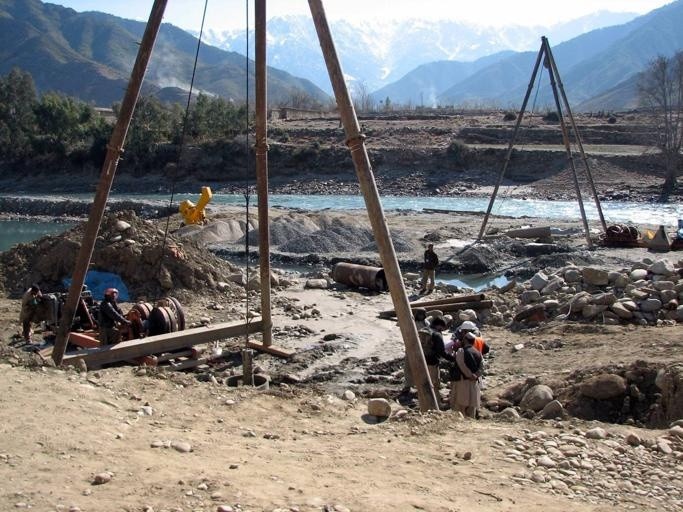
465, 333, 477, 341
460, 321, 475, 330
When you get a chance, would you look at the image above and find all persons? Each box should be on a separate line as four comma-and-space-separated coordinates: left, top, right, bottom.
18, 283, 42, 345
398, 306, 489, 420
98, 288, 132, 347
422, 243, 438, 292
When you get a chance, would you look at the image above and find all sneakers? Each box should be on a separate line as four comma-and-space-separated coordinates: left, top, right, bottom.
421, 288, 432, 294
441, 403, 448, 409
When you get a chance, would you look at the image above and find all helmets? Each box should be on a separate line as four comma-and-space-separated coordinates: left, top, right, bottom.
105, 288, 118, 300
